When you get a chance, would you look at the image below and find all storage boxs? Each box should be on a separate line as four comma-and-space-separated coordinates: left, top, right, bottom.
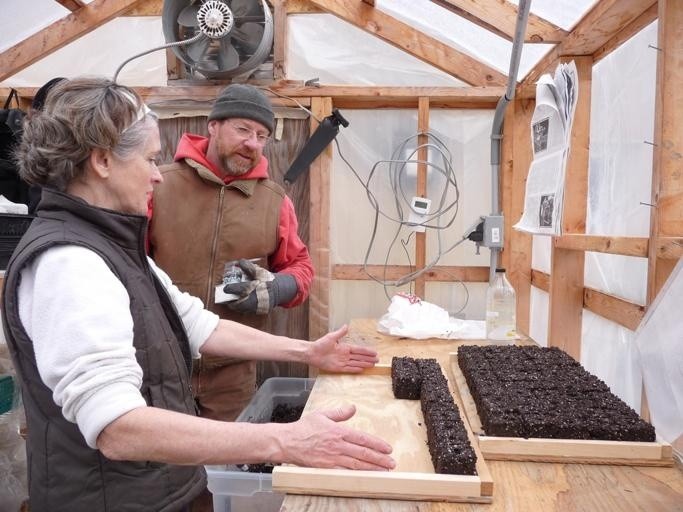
200, 377, 319, 512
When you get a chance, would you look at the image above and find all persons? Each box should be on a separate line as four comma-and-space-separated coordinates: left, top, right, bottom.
144, 86, 319, 422
0, 79, 396, 511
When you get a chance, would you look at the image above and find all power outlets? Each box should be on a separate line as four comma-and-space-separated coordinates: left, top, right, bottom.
474, 214, 505, 250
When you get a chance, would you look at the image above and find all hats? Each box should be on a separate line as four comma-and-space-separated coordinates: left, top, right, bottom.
206, 83, 275, 136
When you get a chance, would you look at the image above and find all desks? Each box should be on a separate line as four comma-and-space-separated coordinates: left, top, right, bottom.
276, 315, 683, 512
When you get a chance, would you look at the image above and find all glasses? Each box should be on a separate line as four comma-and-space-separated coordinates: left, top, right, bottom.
226, 117, 273, 145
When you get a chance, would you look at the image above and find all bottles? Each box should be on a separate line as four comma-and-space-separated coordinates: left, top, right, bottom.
484, 269, 515, 341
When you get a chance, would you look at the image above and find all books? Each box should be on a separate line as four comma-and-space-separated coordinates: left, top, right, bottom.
512, 59, 579, 236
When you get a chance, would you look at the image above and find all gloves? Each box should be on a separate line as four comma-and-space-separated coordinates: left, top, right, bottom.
223, 258, 298, 317
